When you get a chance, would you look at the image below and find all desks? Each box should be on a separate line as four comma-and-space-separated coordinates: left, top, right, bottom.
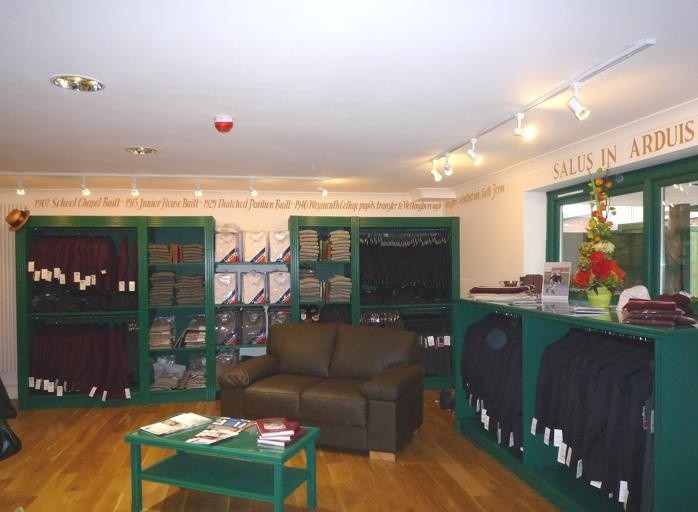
123, 412, 321, 512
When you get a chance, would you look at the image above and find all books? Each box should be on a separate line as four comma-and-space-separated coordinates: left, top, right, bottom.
139, 412, 306, 448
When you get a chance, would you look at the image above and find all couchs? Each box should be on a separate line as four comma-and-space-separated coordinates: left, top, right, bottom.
217, 324, 424, 462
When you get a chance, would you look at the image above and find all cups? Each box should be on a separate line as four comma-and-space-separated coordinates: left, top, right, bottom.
498, 280, 518, 288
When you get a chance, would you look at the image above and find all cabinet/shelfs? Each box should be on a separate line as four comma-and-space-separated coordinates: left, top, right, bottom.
449, 291, 697, 511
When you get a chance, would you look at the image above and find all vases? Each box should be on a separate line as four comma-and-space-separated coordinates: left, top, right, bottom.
586, 284, 612, 308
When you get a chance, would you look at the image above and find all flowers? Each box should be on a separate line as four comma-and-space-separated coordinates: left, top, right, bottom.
571, 168, 626, 297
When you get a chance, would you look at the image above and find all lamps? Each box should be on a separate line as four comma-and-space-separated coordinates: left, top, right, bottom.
429, 80, 591, 182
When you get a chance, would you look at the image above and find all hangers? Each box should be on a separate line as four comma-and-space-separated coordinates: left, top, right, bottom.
32, 316, 138, 342
544, 326, 655, 378
359, 227, 448, 250
467, 311, 521, 338
27, 231, 137, 250
